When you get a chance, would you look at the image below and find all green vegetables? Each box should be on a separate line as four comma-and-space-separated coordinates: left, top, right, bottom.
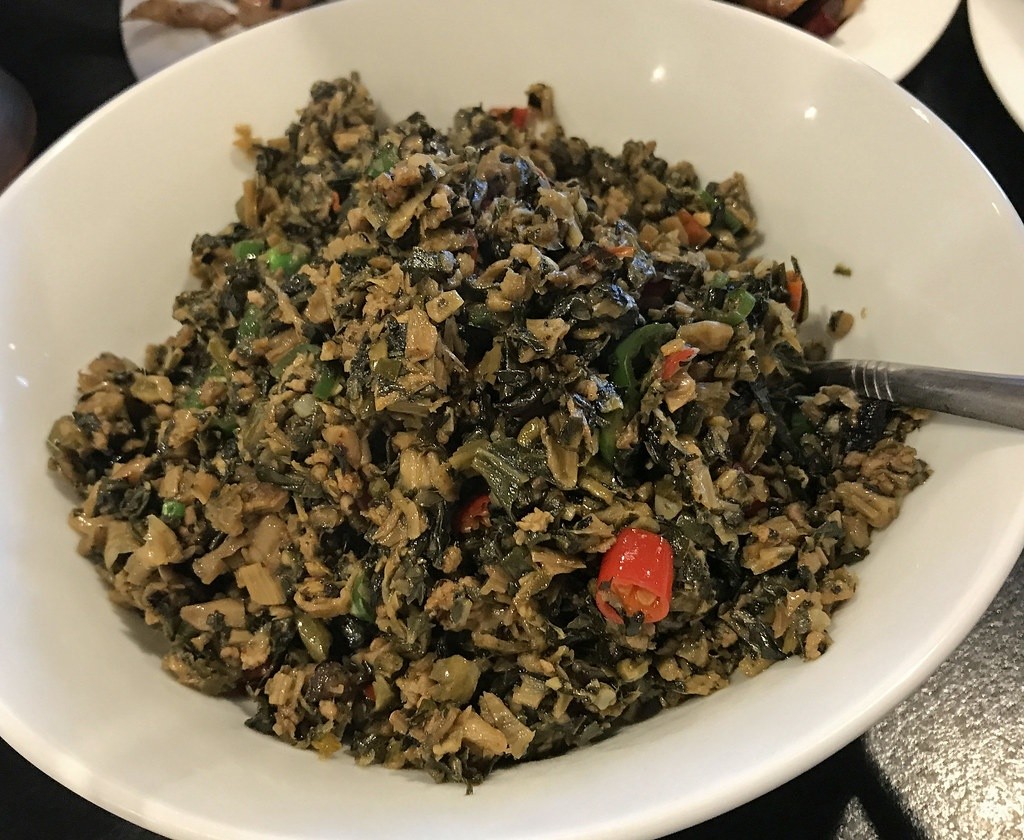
42, 71, 937, 796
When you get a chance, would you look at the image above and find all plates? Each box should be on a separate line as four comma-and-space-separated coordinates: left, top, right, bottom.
120, 0, 957, 85
0, 0, 1024, 840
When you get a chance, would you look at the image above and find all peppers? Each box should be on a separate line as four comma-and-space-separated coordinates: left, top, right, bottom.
595, 529, 674, 624
